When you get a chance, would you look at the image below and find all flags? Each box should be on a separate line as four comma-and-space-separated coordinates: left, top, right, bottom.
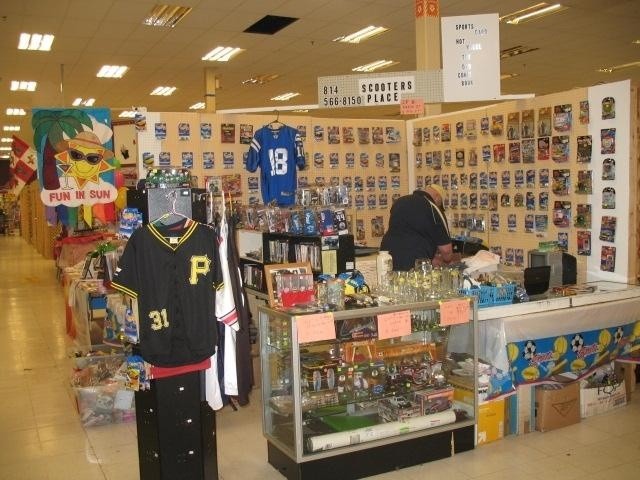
6, 136, 38, 198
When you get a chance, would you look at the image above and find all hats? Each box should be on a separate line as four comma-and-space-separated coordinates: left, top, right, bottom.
55, 131, 115, 162
427, 183, 446, 212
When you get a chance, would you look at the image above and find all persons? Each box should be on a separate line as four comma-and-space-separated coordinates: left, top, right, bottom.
379, 184, 454, 272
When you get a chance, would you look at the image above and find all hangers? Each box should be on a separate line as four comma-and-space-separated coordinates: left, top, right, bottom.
266, 111, 285, 131
152, 191, 193, 229
205, 188, 236, 220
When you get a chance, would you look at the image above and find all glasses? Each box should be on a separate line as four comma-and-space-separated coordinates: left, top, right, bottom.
66, 147, 104, 165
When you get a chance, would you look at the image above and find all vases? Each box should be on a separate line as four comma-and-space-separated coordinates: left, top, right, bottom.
375, 250, 393, 294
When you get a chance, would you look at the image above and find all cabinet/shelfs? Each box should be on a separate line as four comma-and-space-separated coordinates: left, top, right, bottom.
258, 288, 479, 480
17, 181, 61, 260
235, 227, 357, 358
447, 280, 639, 436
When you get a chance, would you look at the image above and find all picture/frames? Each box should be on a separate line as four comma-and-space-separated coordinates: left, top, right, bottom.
264, 260, 316, 310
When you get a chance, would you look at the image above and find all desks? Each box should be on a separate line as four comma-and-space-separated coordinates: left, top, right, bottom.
63, 267, 104, 352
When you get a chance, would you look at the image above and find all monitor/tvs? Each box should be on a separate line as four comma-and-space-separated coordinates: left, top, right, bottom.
528, 250, 577, 289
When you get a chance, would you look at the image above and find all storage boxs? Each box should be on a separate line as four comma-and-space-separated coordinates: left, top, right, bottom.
68, 343, 135, 427
442, 355, 640, 449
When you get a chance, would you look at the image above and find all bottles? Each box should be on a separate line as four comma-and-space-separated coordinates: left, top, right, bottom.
375, 249, 393, 287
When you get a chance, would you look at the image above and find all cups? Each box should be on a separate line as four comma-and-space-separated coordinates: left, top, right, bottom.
379, 258, 464, 303
